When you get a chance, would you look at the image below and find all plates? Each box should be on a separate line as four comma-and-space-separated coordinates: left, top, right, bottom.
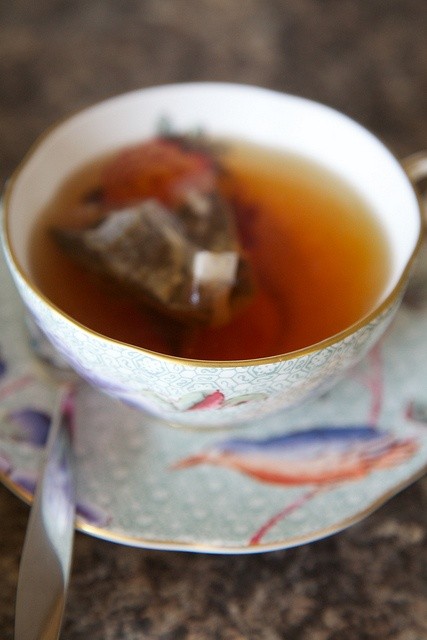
2, 239, 426, 554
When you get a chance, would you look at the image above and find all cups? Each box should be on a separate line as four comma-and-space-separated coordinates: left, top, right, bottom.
1, 79, 422, 432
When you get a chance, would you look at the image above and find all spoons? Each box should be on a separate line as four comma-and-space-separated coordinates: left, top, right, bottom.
17, 311, 74, 633
